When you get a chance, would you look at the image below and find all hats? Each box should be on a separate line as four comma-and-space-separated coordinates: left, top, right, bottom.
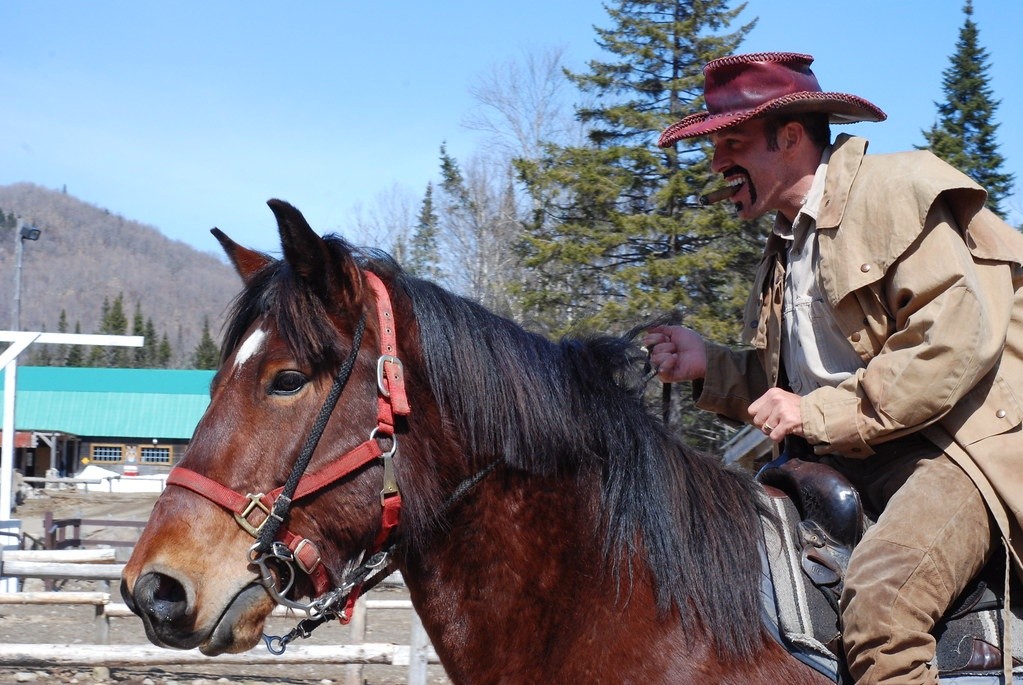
658, 52, 887, 150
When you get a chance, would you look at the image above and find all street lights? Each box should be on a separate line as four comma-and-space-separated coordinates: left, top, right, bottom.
9, 214, 42, 332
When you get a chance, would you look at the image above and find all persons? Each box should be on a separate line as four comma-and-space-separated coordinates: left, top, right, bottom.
644, 53, 1023, 685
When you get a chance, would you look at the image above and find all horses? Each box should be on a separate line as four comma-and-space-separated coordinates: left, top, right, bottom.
120, 198, 1023, 684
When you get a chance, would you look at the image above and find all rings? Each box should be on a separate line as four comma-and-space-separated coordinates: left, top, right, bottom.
764, 421, 774, 432
